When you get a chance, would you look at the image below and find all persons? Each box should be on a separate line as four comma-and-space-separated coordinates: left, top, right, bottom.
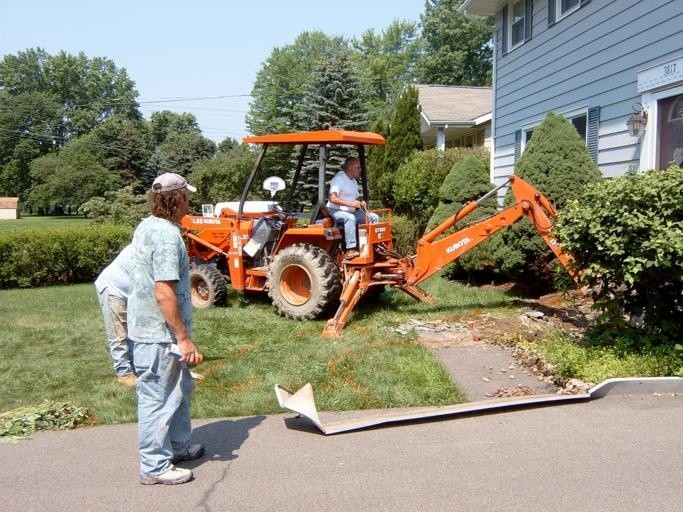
324, 156, 379, 260
124, 171, 205, 486
93, 238, 146, 387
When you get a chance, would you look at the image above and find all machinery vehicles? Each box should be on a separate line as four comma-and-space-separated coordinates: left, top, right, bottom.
180, 129, 601, 337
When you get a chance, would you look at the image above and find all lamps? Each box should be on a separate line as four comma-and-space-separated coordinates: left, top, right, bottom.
625, 102, 648, 138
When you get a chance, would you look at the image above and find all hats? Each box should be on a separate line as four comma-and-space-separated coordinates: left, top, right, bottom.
151, 172, 197, 194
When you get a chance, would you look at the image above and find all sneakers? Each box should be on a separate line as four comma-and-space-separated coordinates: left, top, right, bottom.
140, 466, 193, 485
171, 443, 205, 464
117, 373, 138, 387
346, 249, 359, 256
